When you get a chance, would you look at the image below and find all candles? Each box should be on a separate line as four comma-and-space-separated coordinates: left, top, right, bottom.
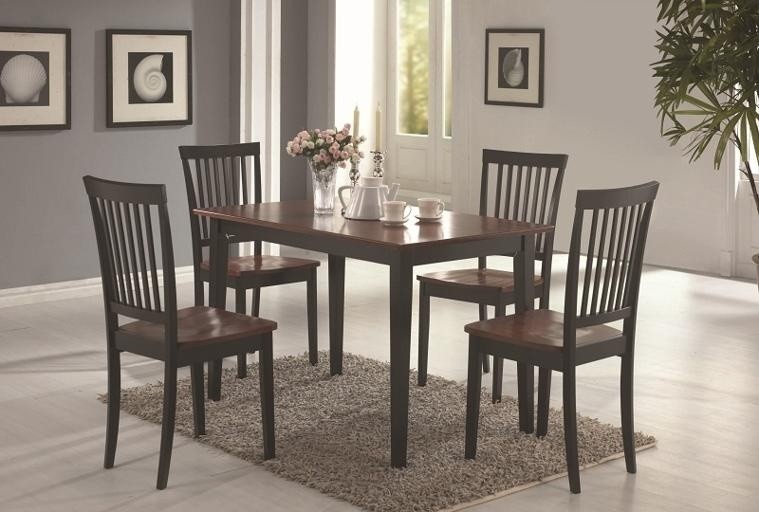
353, 106, 361, 169
375, 106, 383, 151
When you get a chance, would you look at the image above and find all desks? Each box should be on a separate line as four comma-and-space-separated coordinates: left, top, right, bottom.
193, 200, 555, 469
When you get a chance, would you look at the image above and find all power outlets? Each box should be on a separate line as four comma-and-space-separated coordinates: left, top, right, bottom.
747, 245, 759, 268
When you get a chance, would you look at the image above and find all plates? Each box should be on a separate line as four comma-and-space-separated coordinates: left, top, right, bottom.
415, 216, 444, 222
379, 218, 408, 226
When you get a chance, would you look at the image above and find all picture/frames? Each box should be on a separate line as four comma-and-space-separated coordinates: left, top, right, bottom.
107, 29, 193, 128
484, 28, 546, 108
0, 26, 74, 133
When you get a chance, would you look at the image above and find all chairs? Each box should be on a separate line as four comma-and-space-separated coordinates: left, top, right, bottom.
464, 180, 661, 494
417, 149, 570, 403
83, 175, 280, 491
178, 142, 321, 379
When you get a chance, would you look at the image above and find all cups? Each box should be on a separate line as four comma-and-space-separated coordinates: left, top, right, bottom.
382, 201, 412, 219
417, 197, 445, 217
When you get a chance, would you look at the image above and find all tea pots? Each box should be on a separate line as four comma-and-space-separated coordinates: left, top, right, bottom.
338, 177, 401, 221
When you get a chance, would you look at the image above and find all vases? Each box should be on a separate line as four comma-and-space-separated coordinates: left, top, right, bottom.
308, 161, 337, 215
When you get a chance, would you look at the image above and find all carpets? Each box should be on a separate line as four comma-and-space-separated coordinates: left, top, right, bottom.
97, 348, 658, 512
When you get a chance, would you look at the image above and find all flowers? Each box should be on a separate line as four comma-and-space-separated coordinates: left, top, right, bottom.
286, 123, 366, 169
